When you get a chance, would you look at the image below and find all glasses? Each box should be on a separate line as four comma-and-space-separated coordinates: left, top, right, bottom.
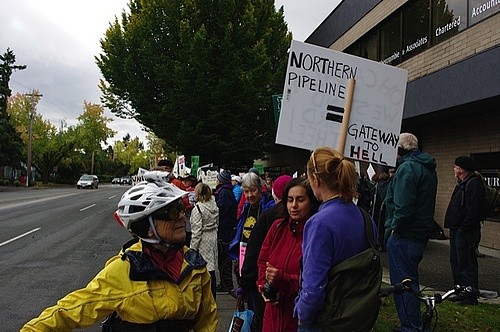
152, 203, 186, 222
454, 167, 460, 170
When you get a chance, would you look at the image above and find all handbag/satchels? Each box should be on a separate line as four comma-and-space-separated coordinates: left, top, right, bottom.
227, 298, 254, 332
311, 207, 383, 332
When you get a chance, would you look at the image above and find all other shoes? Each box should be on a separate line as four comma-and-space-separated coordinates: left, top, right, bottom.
450, 294, 478, 306
216, 284, 235, 294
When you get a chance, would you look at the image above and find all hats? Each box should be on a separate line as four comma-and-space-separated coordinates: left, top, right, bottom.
217, 170, 232, 185
272, 175, 292, 199
454, 155, 476, 174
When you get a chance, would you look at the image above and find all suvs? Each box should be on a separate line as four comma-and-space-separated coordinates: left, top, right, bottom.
120, 176, 133, 185
77, 174, 99, 189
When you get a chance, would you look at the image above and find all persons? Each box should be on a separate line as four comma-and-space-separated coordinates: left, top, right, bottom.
19, 179, 219, 332
379, 133, 438, 332
355, 169, 395, 229
169, 165, 323, 332
293, 147, 383, 332
443, 155, 487, 304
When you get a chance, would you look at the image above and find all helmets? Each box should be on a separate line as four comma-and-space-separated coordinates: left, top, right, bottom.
114, 179, 191, 230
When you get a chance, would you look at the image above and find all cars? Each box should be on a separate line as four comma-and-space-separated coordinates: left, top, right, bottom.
111, 178, 120, 184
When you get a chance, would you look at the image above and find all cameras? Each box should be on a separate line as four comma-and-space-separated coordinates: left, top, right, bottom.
263, 282, 279, 302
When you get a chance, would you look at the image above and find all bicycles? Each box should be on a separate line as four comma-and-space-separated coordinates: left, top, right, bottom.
379, 279, 500, 332
